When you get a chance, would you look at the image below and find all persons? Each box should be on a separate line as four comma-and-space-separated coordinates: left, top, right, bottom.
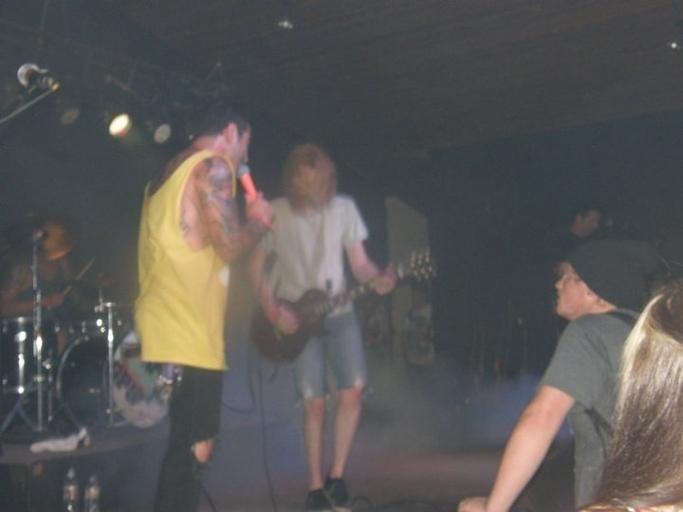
250, 145, 396, 511
561, 205, 603, 240
579, 279, 683, 512
458, 226, 661, 512
135, 100, 276, 512
1, 216, 75, 316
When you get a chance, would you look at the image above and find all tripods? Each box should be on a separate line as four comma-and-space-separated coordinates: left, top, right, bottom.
89, 306, 137, 443
1, 294, 84, 447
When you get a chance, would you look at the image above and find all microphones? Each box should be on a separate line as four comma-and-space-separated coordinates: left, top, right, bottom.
240, 163, 274, 228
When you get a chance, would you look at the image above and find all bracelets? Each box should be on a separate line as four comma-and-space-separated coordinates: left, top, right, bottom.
44, 294, 52, 310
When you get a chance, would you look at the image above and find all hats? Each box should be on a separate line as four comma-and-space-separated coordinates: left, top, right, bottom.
568, 240, 670, 312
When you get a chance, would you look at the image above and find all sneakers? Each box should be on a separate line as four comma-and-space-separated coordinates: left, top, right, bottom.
307, 476, 349, 512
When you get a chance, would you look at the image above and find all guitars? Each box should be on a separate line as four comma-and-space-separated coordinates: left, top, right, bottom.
250, 248, 438, 365
2, 261, 117, 317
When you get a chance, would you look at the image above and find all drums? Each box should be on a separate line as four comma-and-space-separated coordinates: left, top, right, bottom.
69, 310, 127, 346
0, 315, 59, 394
56, 321, 175, 439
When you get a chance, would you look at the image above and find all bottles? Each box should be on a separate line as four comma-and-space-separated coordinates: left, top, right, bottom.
83, 475, 101, 511
61, 468, 78, 512
